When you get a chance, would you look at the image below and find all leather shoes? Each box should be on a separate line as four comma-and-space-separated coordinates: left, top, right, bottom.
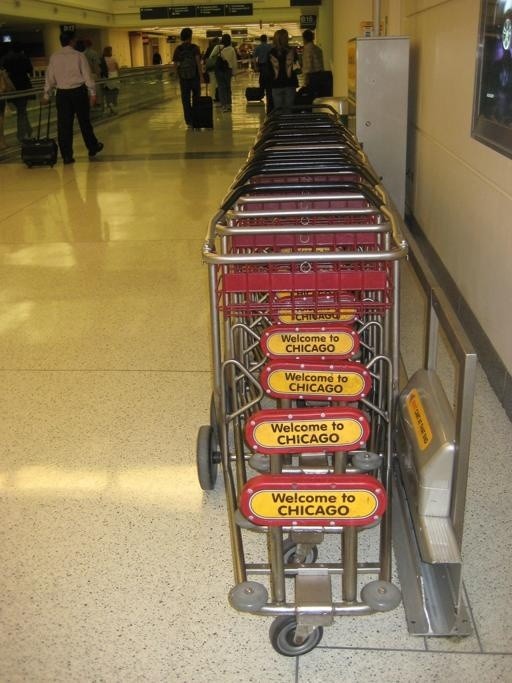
88, 142, 104, 156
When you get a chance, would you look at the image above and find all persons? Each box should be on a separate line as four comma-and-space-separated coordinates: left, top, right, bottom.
80, 39, 101, 109
205, 37, 219, 60
208, 34, 237, 112
103, 46, 122, 117
153, 49, 161, 65
252, 35, 275, 98
2, 44, 34, 141
41, 31, 105, 165
173, 28, 205, 131
303, 29, 323, 84
273, 29, 299, 108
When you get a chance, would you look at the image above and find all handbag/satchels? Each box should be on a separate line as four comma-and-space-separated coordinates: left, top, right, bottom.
206, 44, 222, 71
295, 85, 316, 114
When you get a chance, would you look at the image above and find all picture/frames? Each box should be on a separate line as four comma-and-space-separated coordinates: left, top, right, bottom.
469, 0, 512, 160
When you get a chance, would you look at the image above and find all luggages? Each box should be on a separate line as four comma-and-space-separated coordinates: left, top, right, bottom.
20, 99, 59, 169
191, 81, 214, 130
245, 65, 267, 104
